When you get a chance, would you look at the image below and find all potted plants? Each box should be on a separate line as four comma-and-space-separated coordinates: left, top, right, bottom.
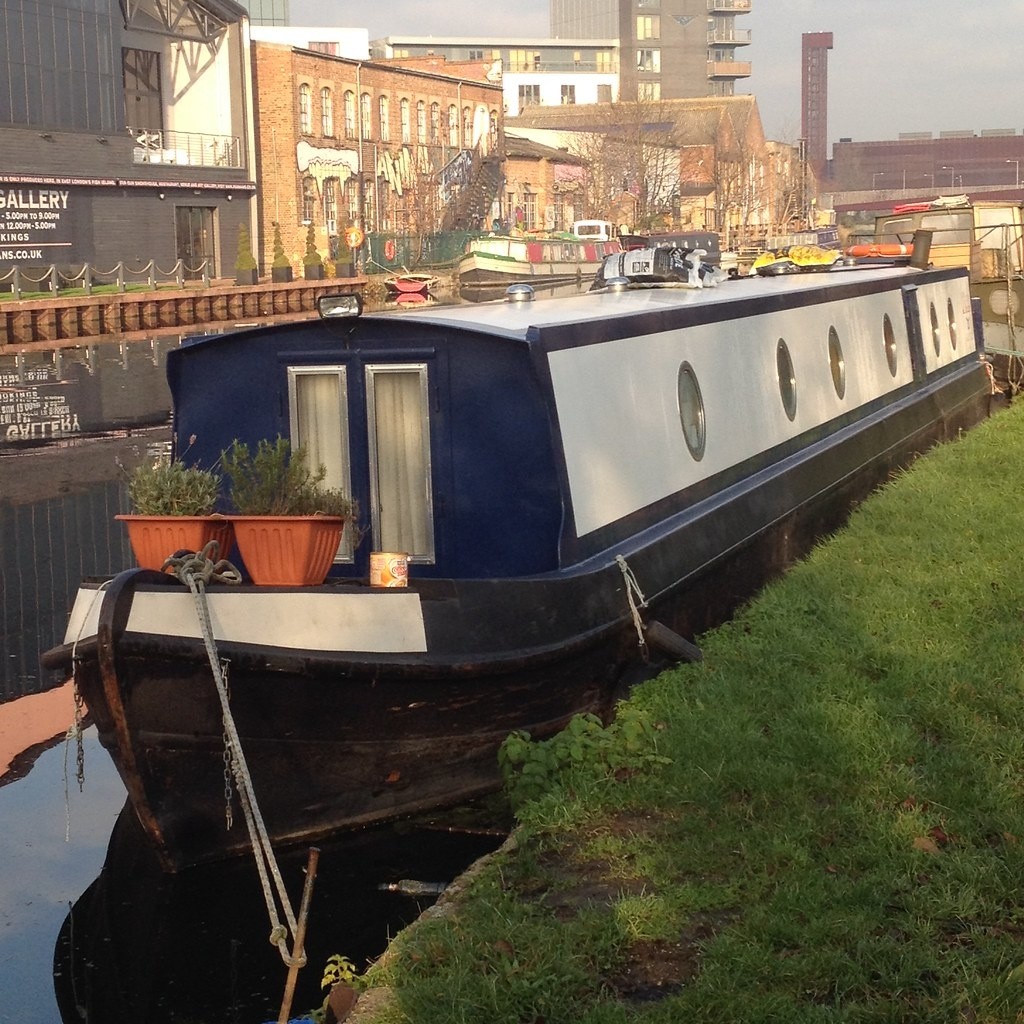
234, 223, 259, 286
336, 218, 354, 278
302, 220, 325, 280
115, 432, 239, 575
270, 222, 292, 282
222, 434, 372, 587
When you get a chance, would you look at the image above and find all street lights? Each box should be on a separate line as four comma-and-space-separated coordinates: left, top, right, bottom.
1005, 160, 1019, 185
873, 172, 884, 190
923, 174, 934, 188
955, 175, 962, 187
942, 166, 954, 187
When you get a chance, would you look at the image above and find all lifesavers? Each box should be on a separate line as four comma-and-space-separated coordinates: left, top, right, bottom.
384, 240, 395, 261
847, 243, 913, 257
344, 226, 364, 248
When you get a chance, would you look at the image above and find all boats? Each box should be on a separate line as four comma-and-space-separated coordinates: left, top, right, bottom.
38, 194, 1024, 886
